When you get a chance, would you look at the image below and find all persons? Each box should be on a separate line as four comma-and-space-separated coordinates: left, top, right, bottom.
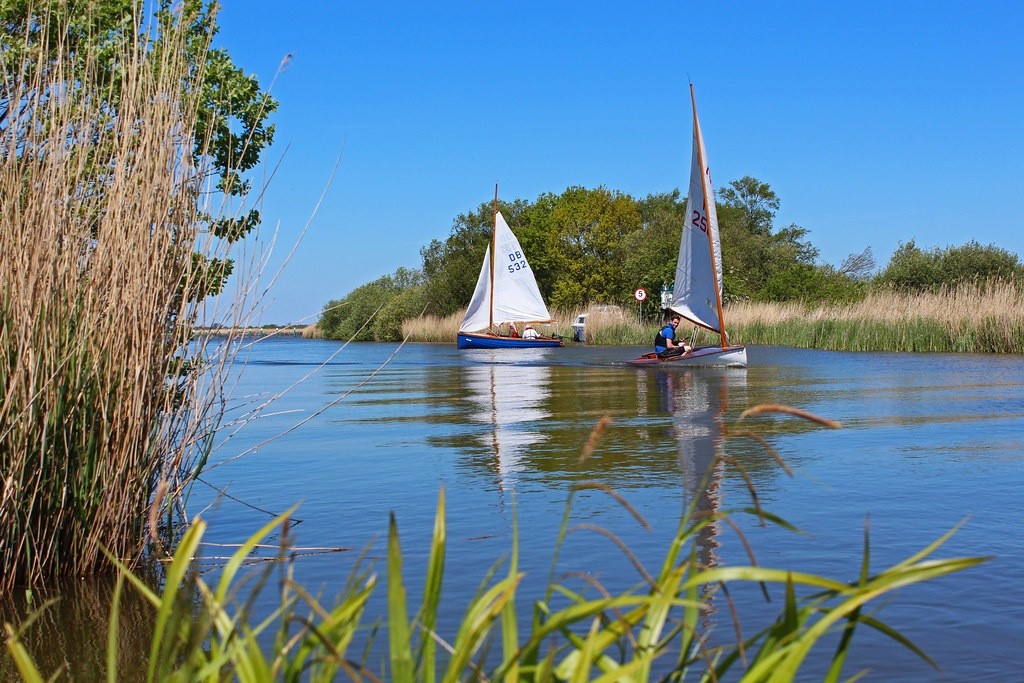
655, 315, 691, 359
522, 324, 542, 339
497, 321, 519, 338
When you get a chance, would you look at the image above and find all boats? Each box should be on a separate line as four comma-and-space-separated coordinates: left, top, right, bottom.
571, 305, 622, 342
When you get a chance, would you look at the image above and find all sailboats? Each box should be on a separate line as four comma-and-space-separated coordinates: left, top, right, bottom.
458, 183, 566, 348
623, 73, 748, 367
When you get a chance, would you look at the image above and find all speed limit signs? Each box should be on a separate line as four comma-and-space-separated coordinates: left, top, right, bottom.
634, 288, 648, 301
